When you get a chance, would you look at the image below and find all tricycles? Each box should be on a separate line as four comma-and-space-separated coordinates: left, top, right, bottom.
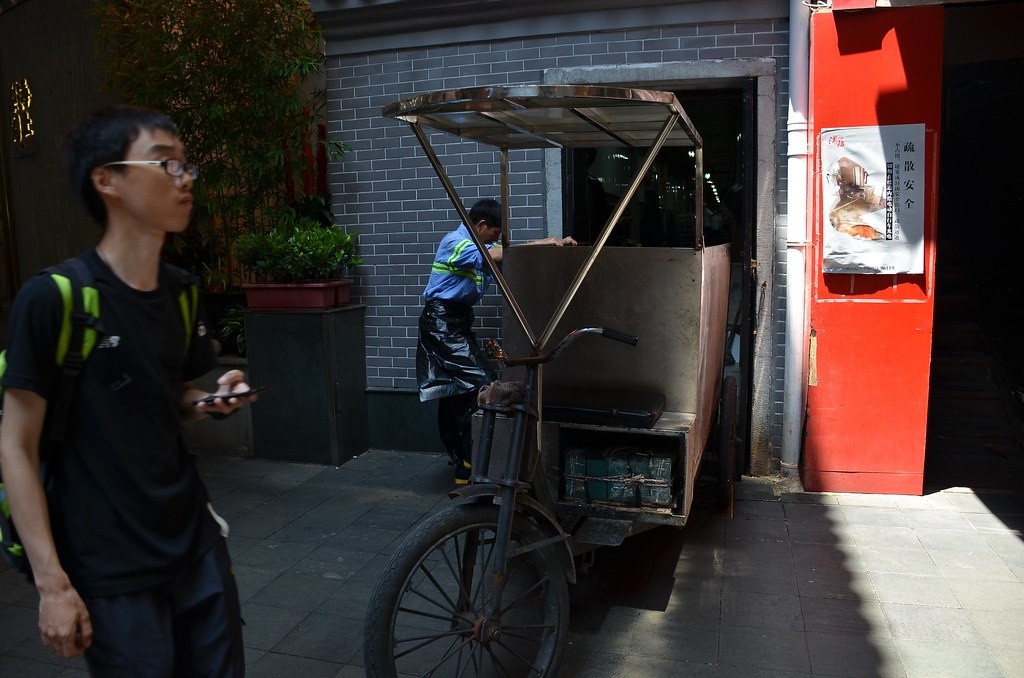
359, 83, 733, 678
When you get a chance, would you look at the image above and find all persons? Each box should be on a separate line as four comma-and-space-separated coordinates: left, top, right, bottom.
417, 199, 578, 486
0, 109, 259, 677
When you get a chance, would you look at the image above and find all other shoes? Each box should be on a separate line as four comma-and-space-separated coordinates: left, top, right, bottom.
455, 463, 471, 484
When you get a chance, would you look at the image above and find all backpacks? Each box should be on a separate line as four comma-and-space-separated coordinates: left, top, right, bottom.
0, 258, 198, 573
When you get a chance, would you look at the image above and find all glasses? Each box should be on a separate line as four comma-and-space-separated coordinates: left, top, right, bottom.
101, 158, 199, 180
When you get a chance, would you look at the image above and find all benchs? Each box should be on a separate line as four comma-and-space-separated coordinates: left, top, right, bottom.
505, 382, 668, 430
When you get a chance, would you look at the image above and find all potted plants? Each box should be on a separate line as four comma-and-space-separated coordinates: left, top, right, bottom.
232, 222, 362, 308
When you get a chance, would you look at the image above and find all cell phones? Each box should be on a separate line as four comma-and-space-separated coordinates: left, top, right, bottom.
192, 386, 272, 406
